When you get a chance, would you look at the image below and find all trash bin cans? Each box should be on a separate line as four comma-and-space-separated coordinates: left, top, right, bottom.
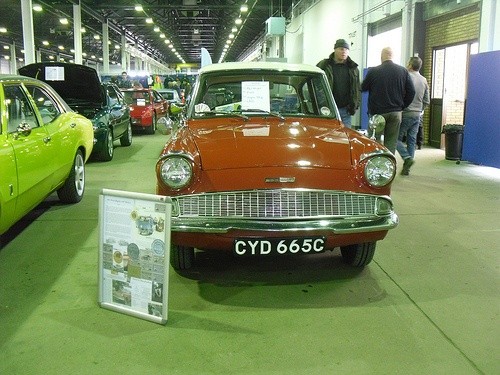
442, 124, 464, 160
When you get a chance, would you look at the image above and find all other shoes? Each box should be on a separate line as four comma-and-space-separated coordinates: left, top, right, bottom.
401, 158, 414, 174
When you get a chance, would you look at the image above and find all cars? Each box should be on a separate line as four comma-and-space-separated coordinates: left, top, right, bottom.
122, 87, 183, 134
14, 62, 134, 162
155, 61, 400, 271
0, 73, 95, 235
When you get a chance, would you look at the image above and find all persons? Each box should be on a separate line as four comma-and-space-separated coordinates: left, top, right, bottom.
168, 79, 193, 99
396, 56, 429, 175
360, 45, 415, 155
315, 38, 360, 129
119, 72, 132, 88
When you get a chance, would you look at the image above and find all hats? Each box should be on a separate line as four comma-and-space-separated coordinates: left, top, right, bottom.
335, 39, 349, 49
